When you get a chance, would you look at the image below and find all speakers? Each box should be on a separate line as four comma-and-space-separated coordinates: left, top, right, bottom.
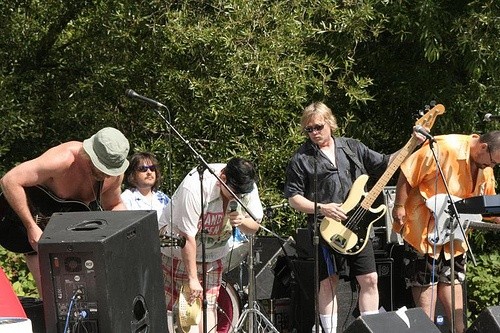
294, 258, 340, 333
351, 258, 413, 311
465, 306, 500, 333
37, 210, 171, 333
344, 306, 442, 333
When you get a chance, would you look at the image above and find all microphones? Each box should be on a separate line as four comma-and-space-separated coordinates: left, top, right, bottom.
125, 89, 164, 108
484, 113, 500, 120
230, 201, 238, 240
412, 124, 437, 143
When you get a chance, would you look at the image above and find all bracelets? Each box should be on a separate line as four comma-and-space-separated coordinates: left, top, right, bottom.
317, 204, 321, 215
394, 205, 405, 207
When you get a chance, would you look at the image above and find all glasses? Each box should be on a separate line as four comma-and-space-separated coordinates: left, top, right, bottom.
135, 164, 157, 172
304, 120, 329, 133
488, 144, 500, 167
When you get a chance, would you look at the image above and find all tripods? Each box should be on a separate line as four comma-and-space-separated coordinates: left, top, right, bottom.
231, 236, 282, 333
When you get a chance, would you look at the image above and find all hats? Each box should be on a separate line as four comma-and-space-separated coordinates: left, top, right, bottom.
178, 284, 201, 333
83, 127, 129, 176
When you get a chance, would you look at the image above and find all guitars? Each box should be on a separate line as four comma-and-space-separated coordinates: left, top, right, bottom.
319, 100, 445, 255
0, 183, 187, 254
424, 193, 500, 246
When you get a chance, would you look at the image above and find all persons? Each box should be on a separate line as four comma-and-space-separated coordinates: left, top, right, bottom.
120, 152, 170, 221
158, 157, 264, 333
0, 126, 131, 333
284, 101, 430, 333
391, 130, 500, 333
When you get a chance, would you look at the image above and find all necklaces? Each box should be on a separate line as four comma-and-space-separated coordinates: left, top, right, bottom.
144, 200, 153, 209
